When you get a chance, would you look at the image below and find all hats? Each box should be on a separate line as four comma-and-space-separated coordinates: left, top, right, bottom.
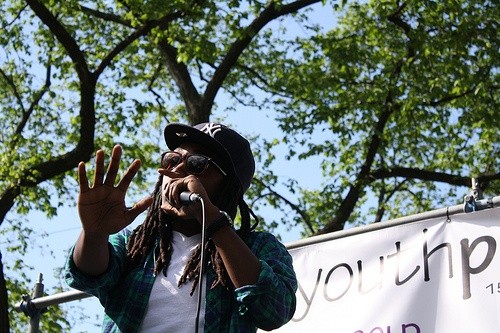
163, 122, 255, 196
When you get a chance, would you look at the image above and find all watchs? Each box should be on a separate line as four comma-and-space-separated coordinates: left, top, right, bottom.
204, 210, 233, 239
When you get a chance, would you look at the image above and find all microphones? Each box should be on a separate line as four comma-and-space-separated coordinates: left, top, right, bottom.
177, 191, 199, 204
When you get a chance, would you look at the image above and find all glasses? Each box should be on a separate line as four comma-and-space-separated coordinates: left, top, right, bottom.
160, 151, 228, 176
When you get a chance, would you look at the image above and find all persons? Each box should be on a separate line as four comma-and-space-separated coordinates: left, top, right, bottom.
64, 123, 298, 333
